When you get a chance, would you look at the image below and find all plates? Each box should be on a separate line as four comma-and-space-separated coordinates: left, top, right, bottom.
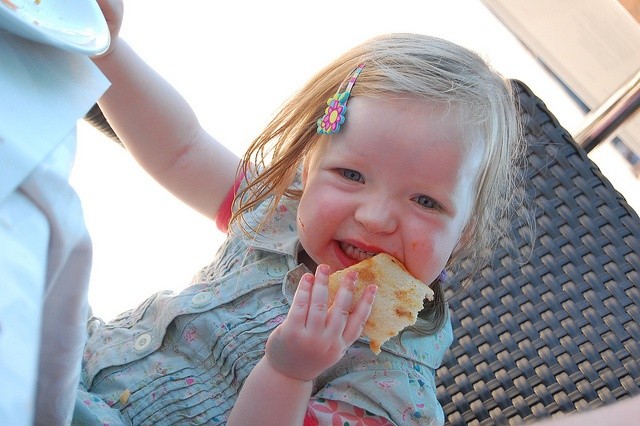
4, 0, 112, 57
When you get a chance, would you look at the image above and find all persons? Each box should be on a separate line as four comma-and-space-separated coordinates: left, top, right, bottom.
74, 1, 512, 426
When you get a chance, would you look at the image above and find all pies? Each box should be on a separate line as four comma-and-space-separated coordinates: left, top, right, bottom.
327, 252, 435, 356
